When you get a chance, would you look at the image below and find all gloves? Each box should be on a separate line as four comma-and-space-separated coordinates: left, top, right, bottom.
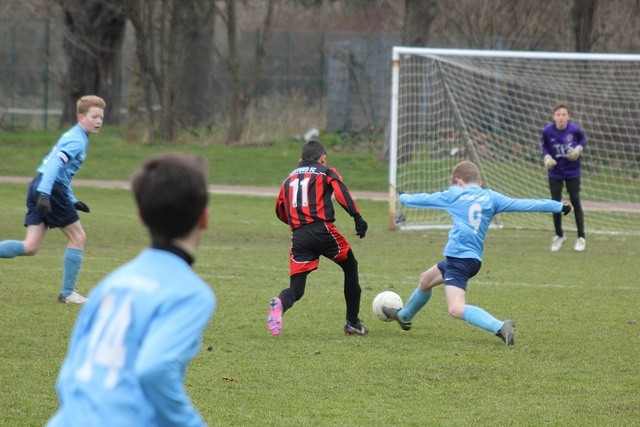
563, 203, 571, 215
34, 195, 52, 216
543, 154, 557, 171
353, 213, 368, 238
567, 144, 584, 162
75, 200, 91, 214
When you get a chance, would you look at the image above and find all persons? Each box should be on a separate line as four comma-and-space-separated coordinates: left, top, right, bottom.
383, 160, 571, 346
0, 95, 106, 304
47, 153, 218, 427
540, 104, 587, 252
266, 141, 369, 336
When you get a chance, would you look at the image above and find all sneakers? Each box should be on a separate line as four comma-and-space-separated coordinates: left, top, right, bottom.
551, 233, 568, 252
574, 236, 586, 252
344, 319, 370, 336
381, 305, 412, 331
57, 290, 87, 305
495, 319, 515, 346
267, 296, 284, 338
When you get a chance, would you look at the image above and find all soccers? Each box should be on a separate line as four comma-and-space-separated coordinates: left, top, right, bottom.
372, 291, 403, 322
